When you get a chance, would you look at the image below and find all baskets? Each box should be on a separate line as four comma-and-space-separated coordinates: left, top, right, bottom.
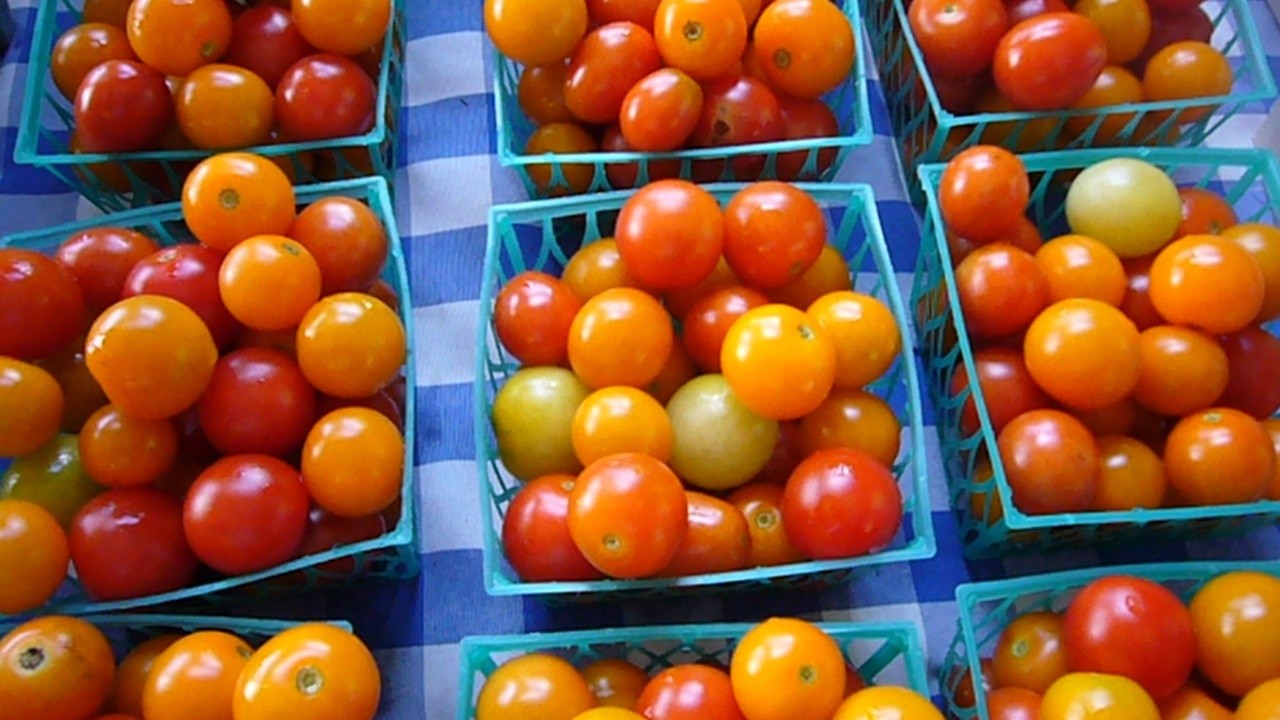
0, 0, 1280, 720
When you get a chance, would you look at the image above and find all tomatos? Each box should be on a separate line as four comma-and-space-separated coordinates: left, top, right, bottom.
0, 0, 1280, 720
